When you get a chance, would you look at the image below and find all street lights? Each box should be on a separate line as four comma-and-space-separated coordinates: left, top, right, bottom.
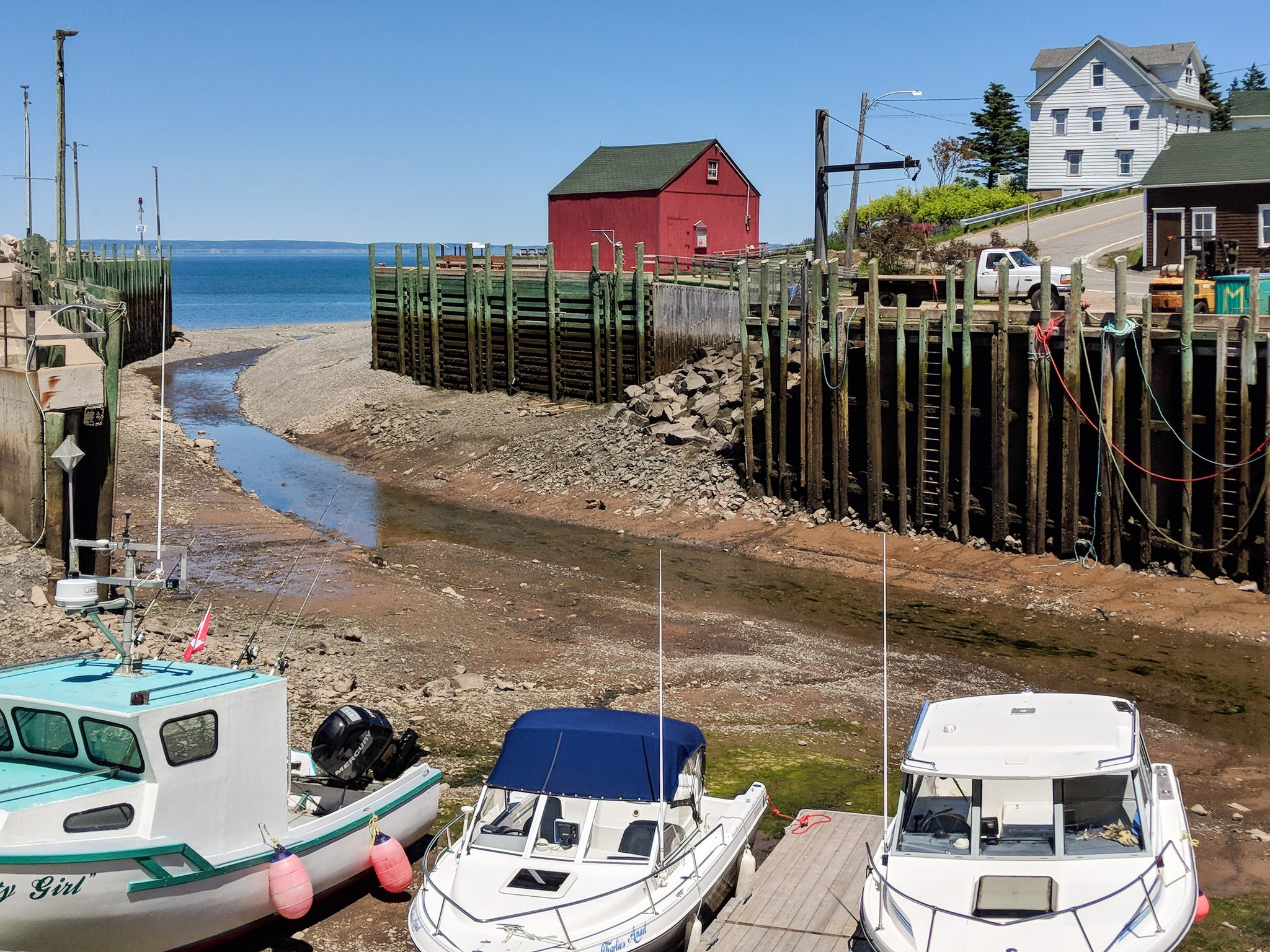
52, 26, 80, 278
843, 89, 922, 271
19, 82, 33, 239
65, 141, 89, 281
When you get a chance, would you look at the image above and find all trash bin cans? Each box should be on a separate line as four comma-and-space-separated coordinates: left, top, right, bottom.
1213, 273, 1270, 317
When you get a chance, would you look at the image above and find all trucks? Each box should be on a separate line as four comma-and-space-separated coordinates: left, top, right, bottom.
837, 247, 1085, 310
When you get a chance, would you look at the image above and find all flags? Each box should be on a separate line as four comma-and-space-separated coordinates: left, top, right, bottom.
183, 604, 212, 662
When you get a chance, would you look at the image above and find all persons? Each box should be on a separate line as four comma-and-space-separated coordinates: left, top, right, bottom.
761, 248, 764, 255
8, 239, 18, 254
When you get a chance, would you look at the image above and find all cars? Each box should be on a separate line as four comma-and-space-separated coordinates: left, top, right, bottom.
858, 217, 931, 251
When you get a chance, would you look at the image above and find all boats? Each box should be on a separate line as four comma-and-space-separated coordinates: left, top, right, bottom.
0, 509, 443, 952
406, 548, 771, 952
857, 531, 1210, 952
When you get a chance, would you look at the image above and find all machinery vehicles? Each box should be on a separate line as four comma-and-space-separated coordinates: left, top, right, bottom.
1148, 234, 1241, 316
